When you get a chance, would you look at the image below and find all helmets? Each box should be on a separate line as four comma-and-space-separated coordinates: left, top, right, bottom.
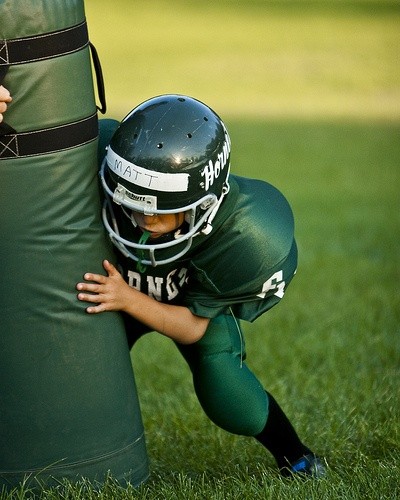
103, 95, 231, 226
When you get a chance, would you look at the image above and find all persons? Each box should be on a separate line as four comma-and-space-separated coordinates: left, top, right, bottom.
0, 83, 330, 485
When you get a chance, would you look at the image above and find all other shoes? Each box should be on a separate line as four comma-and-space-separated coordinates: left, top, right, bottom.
293, 455, 326, 479
